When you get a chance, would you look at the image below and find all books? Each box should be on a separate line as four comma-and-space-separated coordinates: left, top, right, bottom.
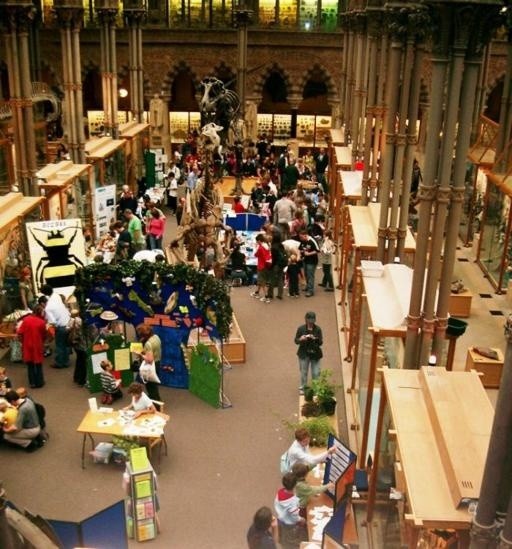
121, 445, 163, 543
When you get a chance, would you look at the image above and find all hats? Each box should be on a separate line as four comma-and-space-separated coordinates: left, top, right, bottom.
305, 312, 316, 322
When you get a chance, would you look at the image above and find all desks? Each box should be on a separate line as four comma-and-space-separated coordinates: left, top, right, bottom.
225, 233, 276, 267
296, 394, 361, 549
76, 407, 169, 476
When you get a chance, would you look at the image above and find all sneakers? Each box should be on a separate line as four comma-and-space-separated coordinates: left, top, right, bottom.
306, 291, 313, 296
26, 441, 38, 452
260, 296, 270, 302
251, 292, 260, 298
102, 393, 112, 404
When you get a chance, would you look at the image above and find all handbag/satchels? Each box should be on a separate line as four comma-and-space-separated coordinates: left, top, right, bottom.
132, 357, 141, 372
280, 451, 289, 474
36, 403, 45, 428
264, 259, 272, 271
9, 340, 23, 362
307, 342, 322, 360
66, 325, 79, 346
139, 360, 161, 384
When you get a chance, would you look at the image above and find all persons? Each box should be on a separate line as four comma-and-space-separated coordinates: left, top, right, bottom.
291, 465, 336, 520
274, 472, 307, 549
411, 160, 424, 198
1, 128, 338, 452
247, 507, 277, 549
281, 428, 337, 488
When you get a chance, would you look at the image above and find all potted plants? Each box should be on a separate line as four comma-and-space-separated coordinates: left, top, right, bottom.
313, 369, 339, 417
300, 385, 318, 417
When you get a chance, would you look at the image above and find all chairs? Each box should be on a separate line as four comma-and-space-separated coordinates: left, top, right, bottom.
137, 399, 168, 457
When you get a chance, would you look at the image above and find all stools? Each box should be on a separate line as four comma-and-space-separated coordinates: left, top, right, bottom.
232, 277, 243, 287
